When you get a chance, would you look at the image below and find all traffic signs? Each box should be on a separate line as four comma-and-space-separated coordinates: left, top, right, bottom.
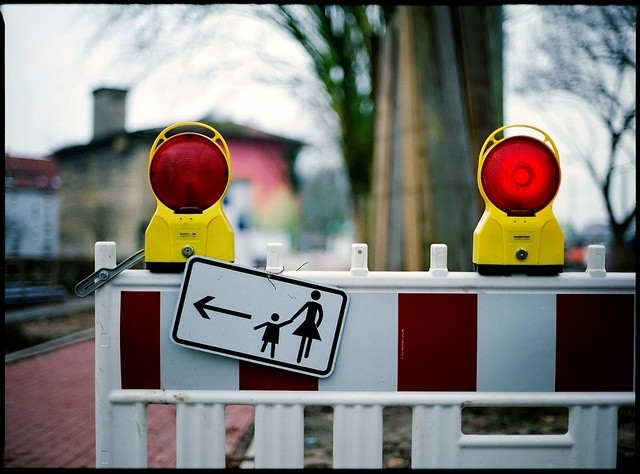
169, 255, 350, 378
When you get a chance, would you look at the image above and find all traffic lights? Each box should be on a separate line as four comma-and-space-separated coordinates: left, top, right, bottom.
472, 124, 565, 276
144, 121, 235, 273
